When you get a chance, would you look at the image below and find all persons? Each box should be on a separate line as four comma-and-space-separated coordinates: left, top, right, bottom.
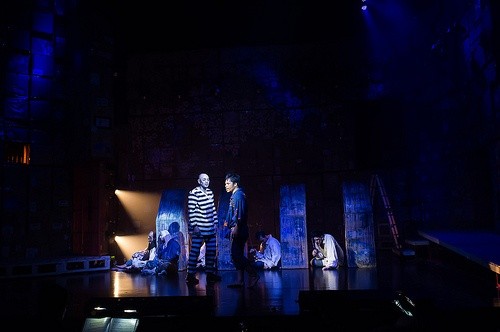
143, 230, 180, 276
186, 173, 221, 283
248, 230, 281, 269
225, 172, 260, 287
116, 232, 156, 271
310, 231, 344, 270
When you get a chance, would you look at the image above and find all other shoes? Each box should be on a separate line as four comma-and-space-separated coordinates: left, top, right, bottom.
185, 272, 199, 283
205, 272, 222, 282
228, 280, 245, 288
247, 272, 260, 288
